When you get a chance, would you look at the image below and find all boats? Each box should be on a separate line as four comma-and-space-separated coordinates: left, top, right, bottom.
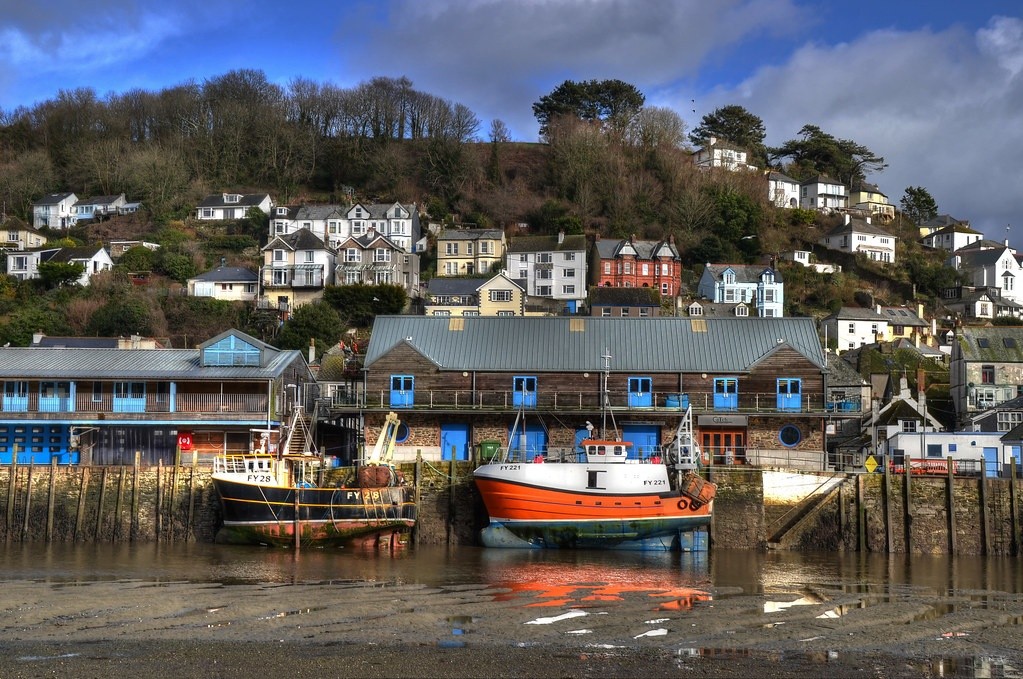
471, 346, 715, 553
211, 382, 421, 552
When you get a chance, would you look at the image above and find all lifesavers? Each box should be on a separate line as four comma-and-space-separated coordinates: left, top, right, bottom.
678, 499, 688, 509
692, 500, 702, 508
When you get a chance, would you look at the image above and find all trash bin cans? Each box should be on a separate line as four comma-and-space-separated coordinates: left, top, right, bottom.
877, 465, 884, 473
331, 458, 340, 467
480, 440, 502, 461
725, 451, 734, 465
